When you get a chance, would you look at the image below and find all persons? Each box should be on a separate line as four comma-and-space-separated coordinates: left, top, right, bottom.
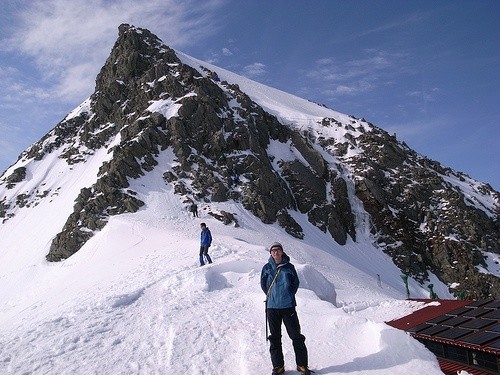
191, 201, 199, 217
260, 242, 313, 375
199, 223, 213, 266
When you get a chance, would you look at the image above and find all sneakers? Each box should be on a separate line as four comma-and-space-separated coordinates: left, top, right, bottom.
272, 366, 284, 375
297, 365, 310, 375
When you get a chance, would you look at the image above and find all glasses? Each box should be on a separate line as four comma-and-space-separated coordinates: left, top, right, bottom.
271, 248, 281, 252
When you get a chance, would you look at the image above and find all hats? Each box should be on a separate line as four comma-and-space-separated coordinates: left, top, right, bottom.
270, 242, 283, 254
